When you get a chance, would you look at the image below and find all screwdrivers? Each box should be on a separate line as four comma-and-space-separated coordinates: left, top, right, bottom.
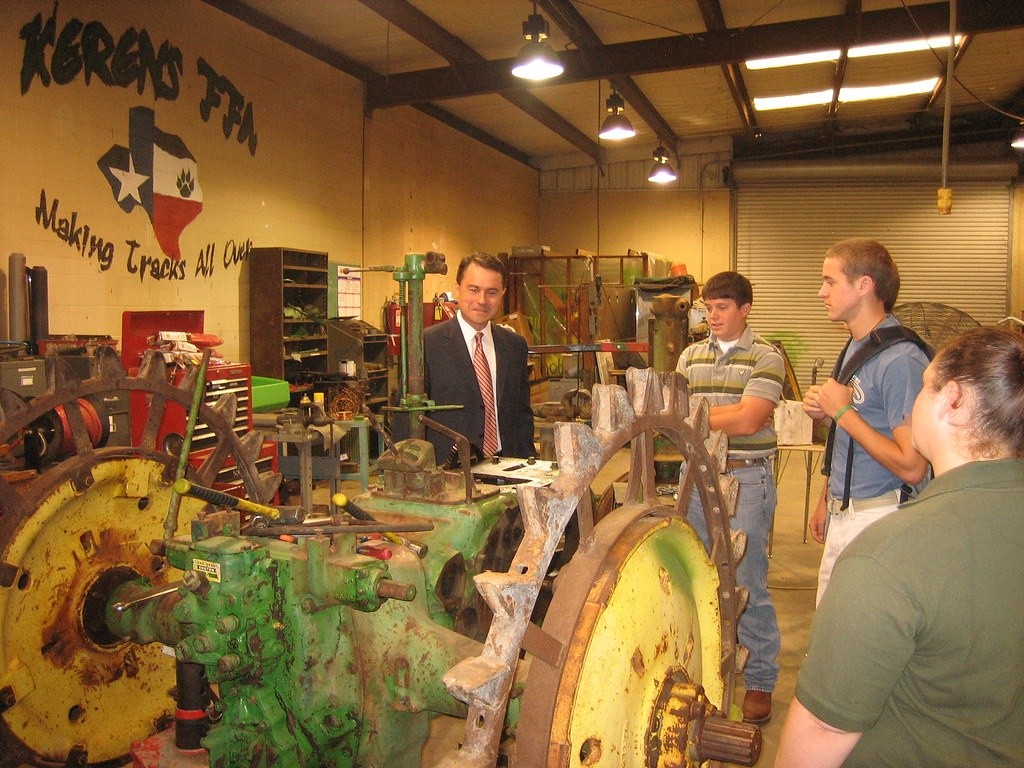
168, 352, 181, 384
356, 545, 393, 560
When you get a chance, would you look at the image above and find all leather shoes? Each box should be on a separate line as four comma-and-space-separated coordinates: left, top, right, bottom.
741, 690, 772, 723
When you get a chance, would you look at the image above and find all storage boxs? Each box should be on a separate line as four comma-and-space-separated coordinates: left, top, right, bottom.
774, 399, 814, 446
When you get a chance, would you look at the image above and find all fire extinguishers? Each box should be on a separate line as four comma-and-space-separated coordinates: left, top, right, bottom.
384, 294, 402, 356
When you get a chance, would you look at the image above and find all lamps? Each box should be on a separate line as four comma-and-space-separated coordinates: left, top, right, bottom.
511, 3, 564, 80
598, 87, 636, 140
648, 134, 677, 183
1010, 120, 1024, 149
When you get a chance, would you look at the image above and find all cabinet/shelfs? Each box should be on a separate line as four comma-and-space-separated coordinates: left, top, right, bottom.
250, 246, 329, 383
326, 319, 389, 415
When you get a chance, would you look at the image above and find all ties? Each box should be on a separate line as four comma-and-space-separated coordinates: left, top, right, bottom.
473, 331, 498, 459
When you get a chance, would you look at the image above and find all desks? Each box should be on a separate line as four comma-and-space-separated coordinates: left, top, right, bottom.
768, 444, 827, 560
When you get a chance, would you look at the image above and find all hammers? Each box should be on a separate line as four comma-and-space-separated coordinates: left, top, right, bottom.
172, 477, 307, 526
332, 493, 430, 561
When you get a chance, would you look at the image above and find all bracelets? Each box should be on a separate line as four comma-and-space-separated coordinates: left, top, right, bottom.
833, 404, 854, 424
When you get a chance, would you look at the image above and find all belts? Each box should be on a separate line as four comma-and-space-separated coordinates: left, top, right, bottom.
827, 490, 899, 515
726, 458, 768, 472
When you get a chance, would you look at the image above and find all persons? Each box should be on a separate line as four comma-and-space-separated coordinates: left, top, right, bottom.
773, 320, 1024, 768
674, 271, 786, 726
802, 236, 935, 613
389, 251, 536, 487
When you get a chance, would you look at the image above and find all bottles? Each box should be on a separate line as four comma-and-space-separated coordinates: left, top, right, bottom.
300, 394, 311, 418
